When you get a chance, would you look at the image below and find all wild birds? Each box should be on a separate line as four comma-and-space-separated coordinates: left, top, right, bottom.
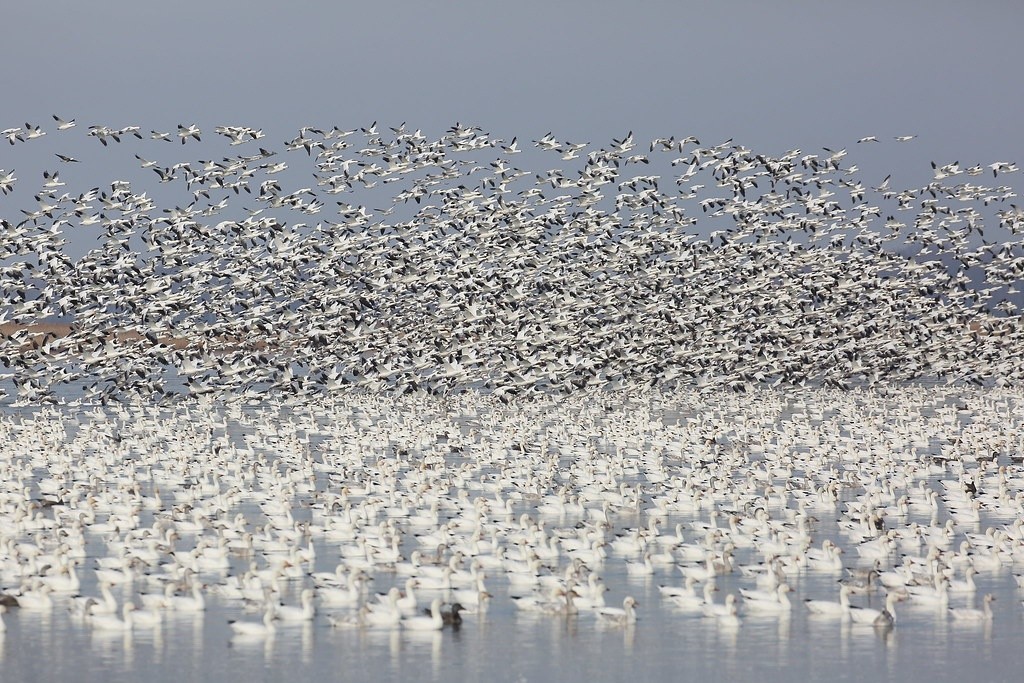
1, 113, 1024, 643
